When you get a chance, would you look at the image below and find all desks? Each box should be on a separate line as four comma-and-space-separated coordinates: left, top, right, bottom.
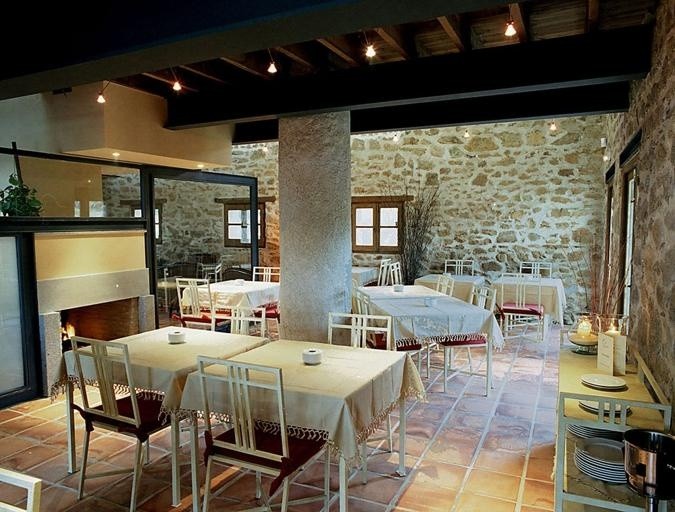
492, 275, 563, 342
58, 322, 270, 504
186, 339, 409, 512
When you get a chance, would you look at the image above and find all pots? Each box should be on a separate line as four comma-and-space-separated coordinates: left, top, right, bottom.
623, 428, 674, 500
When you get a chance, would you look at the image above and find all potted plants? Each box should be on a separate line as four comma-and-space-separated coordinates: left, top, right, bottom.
0, 169, 45, 218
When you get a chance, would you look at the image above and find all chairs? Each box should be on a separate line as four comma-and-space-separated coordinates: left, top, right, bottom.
182, 305, 265, 432
328, 312, 391, 484
70, 336, 201, 512
352, 255, 553, 395
156, 258, 278, 331
196, 355, 330, 511
0, 467, 43, 510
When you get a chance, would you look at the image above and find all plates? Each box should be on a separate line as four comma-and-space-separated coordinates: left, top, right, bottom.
566, 419, 632, 442
574, 438, 628, 484
580, 374, 626, 390
578, 399, 631, 415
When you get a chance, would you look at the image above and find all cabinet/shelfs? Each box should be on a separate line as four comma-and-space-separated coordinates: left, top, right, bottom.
554, 324, 675, 509
